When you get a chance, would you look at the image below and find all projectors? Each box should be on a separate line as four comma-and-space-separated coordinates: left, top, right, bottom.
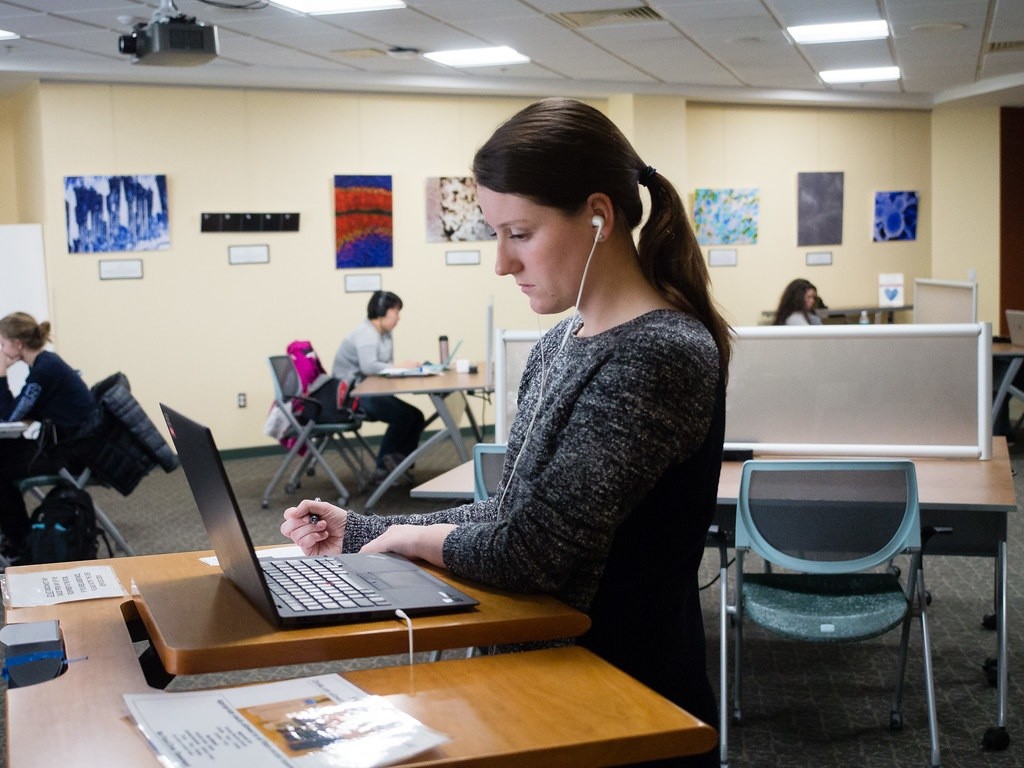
117, 25, 219, 68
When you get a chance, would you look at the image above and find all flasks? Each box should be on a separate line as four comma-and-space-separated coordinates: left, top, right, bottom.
439, 336, 449, 365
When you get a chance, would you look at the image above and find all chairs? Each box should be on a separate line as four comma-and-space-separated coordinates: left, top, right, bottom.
259, 352, 362, 511
283, 339, 385, 487
991, 341, 1024, 436
20, 371, 135, 557
706, 455, 949, 768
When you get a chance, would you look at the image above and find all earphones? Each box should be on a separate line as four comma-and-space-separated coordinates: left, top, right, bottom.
592, 215, 606, 243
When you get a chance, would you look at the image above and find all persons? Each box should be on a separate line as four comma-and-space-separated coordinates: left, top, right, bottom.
280, 101, 733, 728
772, 278, 821, 326
0, 312, 97, 561
334, 290, 423, 488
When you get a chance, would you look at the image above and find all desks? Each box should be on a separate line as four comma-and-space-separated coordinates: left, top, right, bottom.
0, 419, 33, 572
759, 300, 913, 325
128, 551, 597, 687
0, 541, 714, 768
712, 435, 1020, 753
349, 361, 496, 510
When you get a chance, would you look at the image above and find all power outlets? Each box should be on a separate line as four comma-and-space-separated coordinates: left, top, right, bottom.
237, 393, 246, 408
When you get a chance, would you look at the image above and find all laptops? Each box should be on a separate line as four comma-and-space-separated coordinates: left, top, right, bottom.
423, 338, 464, 370
158, 403, 481, 631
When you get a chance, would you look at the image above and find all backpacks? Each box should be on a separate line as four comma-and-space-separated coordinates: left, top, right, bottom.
20, 485, 113, 564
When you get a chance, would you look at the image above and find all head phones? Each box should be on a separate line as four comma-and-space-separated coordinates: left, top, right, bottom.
375, 292, 388, 317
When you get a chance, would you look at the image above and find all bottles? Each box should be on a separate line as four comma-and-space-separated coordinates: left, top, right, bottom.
860, 311, 869, 324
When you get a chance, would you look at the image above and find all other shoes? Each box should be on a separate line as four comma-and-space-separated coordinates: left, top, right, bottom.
383, 453, 417, 487
0, 533, 21, 570
372, 469, 388, 486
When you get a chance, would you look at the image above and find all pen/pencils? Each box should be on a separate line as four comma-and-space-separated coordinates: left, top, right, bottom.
311, 496, 321, 524
